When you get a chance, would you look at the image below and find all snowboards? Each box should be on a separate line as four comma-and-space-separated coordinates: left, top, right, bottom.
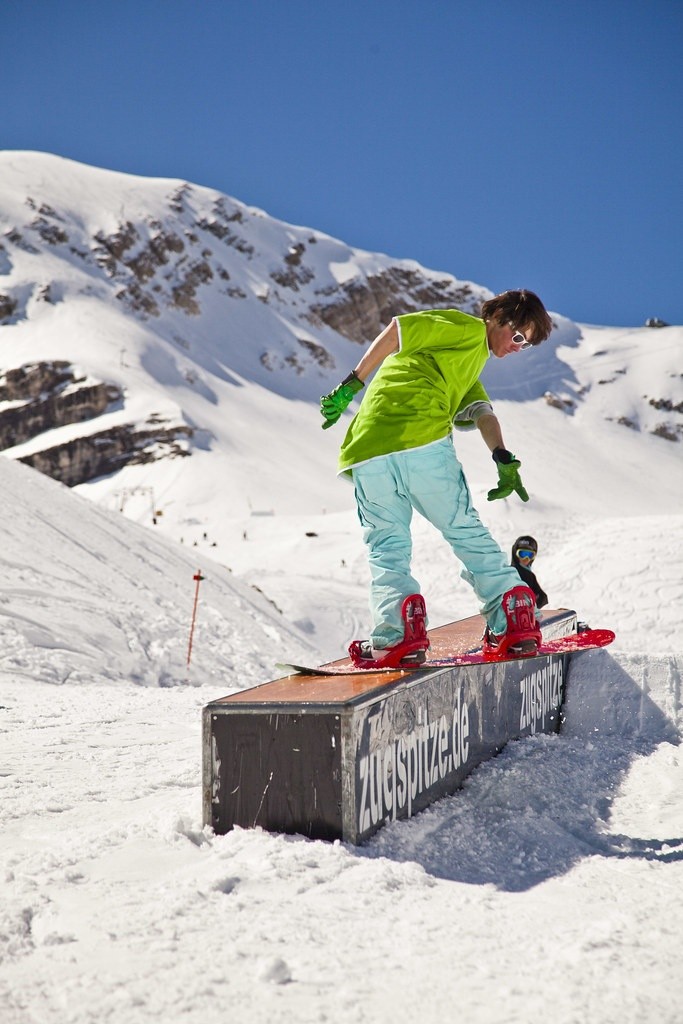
269, 586, 620, 675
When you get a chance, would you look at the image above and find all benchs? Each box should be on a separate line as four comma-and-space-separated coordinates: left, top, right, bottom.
202, 607, 578, 850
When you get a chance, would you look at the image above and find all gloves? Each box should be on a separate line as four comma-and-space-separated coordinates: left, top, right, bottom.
320, 369, 365, 430
487, 447, 529, 502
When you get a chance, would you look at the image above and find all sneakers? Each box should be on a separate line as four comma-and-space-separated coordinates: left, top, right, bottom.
485, 629, 537, 657
348, 639, 427, 664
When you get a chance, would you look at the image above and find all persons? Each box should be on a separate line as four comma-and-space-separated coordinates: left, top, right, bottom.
319, 288, 555, 669
508, 534, 549, 609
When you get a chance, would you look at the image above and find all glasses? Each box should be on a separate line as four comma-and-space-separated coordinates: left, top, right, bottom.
516, 549, 536, 560
506, 316, 532, 350
